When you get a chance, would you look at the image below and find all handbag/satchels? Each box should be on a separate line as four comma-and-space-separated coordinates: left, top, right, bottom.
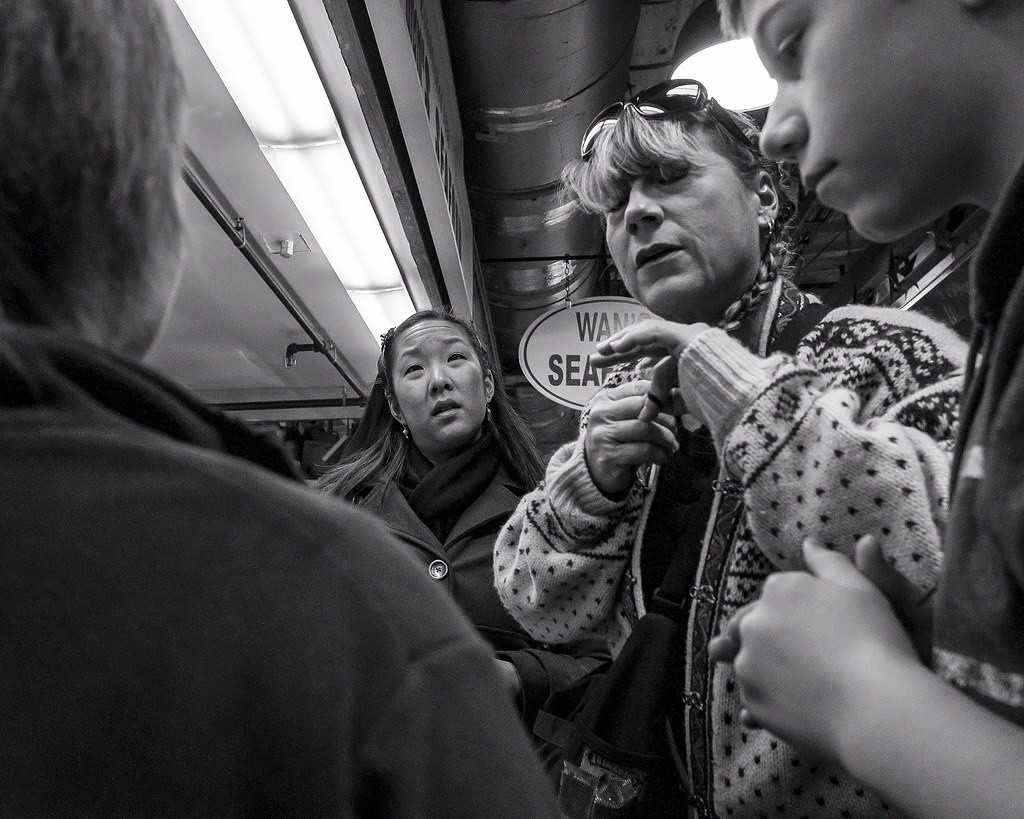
530, 588, 689, 819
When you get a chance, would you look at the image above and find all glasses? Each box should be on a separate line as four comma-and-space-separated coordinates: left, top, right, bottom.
580, 79, 757, 161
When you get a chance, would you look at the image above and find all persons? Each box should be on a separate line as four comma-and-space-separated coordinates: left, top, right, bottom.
1, 0, 571, 819
715, 0, 1024, 819
310, 307, 613, 745
490, 78, 984, 819
